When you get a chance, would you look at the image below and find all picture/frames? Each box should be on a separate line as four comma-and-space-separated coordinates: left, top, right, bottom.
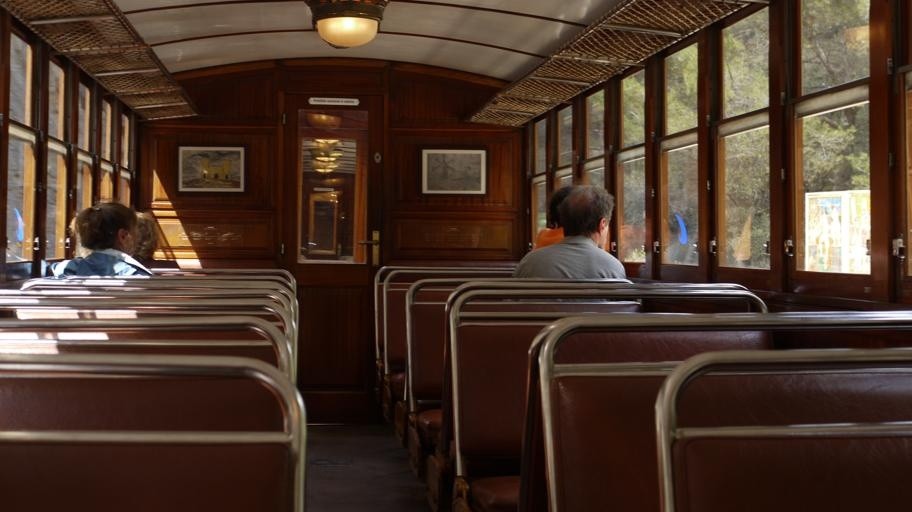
177, 145, 247, 193
422, 148, 487, 195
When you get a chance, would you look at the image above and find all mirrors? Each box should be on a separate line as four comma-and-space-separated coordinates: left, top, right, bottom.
312, 200, 335, 251
295, 106, 370, 266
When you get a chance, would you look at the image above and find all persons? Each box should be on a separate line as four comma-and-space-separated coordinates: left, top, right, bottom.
512, 184, 628, 304
130, 210, 183, 276
529, 185, 566, 249
48, 201, 154, 276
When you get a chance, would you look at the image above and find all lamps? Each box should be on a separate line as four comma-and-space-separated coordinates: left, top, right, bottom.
307, 112, 345, 176
305, 0, 393, 50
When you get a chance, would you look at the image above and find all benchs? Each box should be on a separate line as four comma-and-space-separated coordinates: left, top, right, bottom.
373, 262, 910, 512
0, 265, 310, 512
